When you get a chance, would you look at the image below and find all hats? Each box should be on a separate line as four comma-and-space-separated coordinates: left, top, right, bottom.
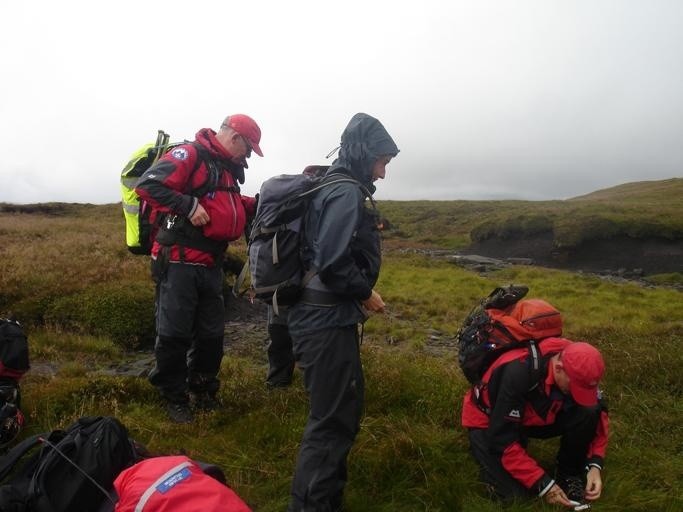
561, 342, 604, 405
224, 115, 265, 157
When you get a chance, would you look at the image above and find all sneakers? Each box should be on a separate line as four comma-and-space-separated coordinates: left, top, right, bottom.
557, 479, 591, 511
162, 396, 192, 424
189, 393, 223, 410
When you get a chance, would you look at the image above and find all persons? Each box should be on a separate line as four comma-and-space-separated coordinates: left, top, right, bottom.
264, 306, 297, 391
456, 334, 612, 512
290, 111, 400, 512
132, 112, 265, 424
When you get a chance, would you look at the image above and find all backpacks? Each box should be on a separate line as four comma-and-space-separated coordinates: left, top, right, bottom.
250, 175, 360, 306
457, 286, 561, 390
1, 416, 252, 512
121, 130, 205, 255
0, 320, 29, 444
